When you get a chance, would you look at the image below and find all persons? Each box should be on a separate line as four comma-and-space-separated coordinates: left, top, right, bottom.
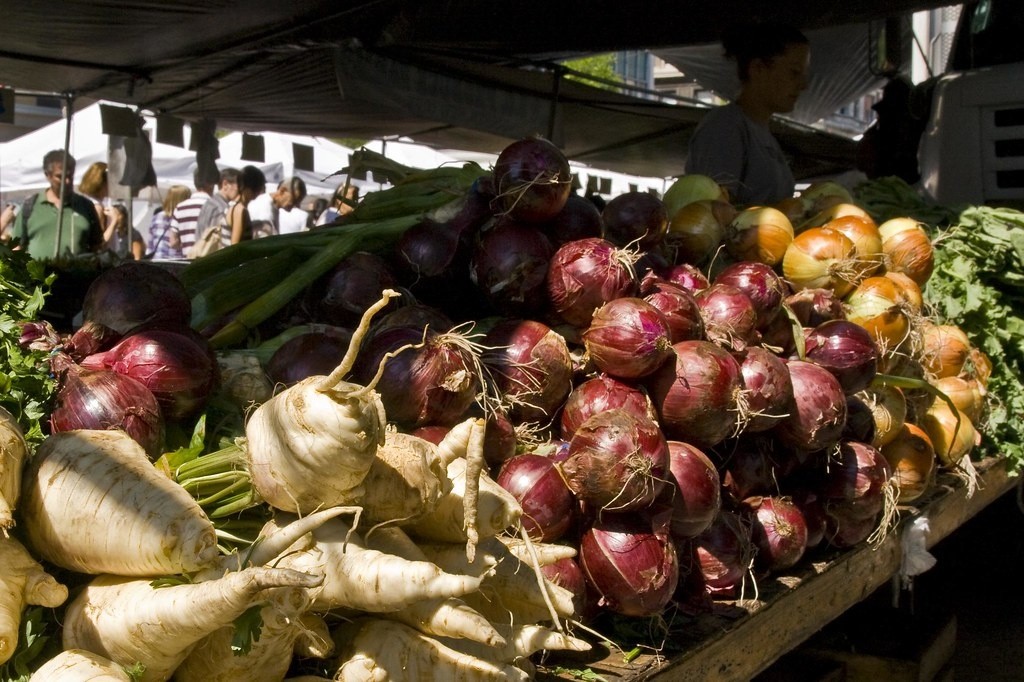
686, 13, 812, 208
146, 166, 359, 260
77, 162, 119, 251
110, 205, 145, 261
853, 79, 930, 184
11, 151, 103, 259
0, 200, 20, 242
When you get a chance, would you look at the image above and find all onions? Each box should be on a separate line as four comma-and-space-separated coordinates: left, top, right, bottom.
28, 137, 990, 617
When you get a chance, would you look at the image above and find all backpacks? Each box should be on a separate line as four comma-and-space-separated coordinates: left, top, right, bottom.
857, 73, 931, 183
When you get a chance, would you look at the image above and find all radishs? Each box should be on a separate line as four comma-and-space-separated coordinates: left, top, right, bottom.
0, 374, 592, 682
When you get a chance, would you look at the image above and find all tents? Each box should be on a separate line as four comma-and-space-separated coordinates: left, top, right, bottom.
219, 131, 392, 201
0, 101, 283, 196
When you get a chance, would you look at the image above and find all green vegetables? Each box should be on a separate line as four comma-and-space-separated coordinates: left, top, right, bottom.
0, 232, 111, 458
836, 174, 1024, 481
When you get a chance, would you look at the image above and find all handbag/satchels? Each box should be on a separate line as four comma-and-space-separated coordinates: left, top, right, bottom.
187, 226, 221, 260
